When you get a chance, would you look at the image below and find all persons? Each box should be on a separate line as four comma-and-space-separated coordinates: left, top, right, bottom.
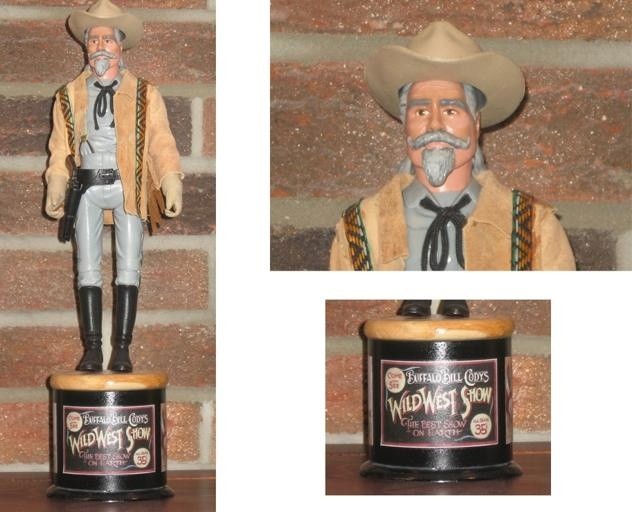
39, 0, 188, 376
323, 19, 580, 272
